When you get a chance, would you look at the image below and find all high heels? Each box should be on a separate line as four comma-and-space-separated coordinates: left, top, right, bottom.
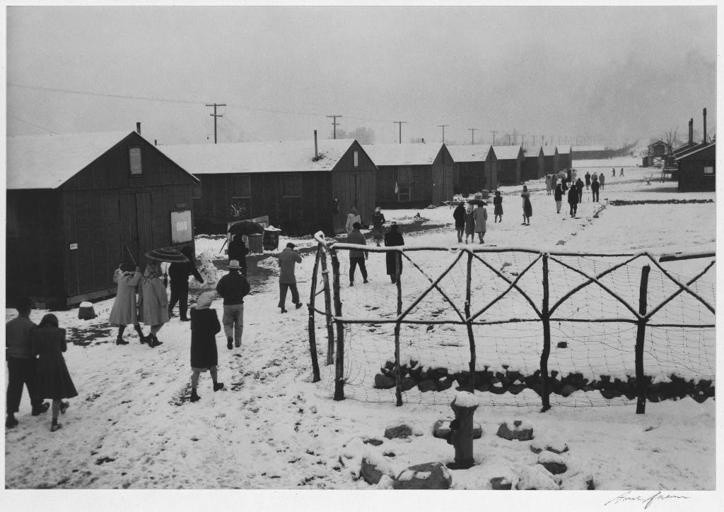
116, 334, 130, 345
140, 336, 149, 344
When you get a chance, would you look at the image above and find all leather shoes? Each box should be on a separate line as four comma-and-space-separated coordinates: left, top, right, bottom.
168, 311, 179, 317
181, 317, 192, 321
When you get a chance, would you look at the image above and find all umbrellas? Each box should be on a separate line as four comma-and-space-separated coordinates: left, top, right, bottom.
143, 245, 190, 271
228, 220, 264, 242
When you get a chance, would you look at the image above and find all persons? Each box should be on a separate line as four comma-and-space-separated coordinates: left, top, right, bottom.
5, 296, 50, 428
276, 242, 302, 313
187, 291, 224, 403
451, 166, 625, 245
28, 313, 77, 432
166, 245, 204, 322
343, 205, 361, 234
226, 232, 250, 281
137, 260, 172, 347
215, 258, 251, 350
382, 221, 406, 286
108, 261, 149, 346
371, 206, 386, 248
345, 221, 369, 287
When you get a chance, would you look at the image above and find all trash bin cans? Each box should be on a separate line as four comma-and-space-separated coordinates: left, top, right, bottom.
248, 233, 263, 253
263, 228, 279, 250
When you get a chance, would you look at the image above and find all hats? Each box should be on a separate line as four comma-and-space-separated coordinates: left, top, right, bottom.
227, 259, 243, 268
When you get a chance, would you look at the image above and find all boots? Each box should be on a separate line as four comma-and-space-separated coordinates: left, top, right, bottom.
213, 381, 224, 392
191, 388, 201, 402
147, 333, 163, 347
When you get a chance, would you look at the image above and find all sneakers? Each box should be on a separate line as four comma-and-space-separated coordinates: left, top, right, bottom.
228, 337, 234, 349
281, 309, 287, 313
6, 419, 19, 428
52, 424, 62, 432
62, 401, 69, 414
32, 402, 50, 415
297, 303, 302, 309
